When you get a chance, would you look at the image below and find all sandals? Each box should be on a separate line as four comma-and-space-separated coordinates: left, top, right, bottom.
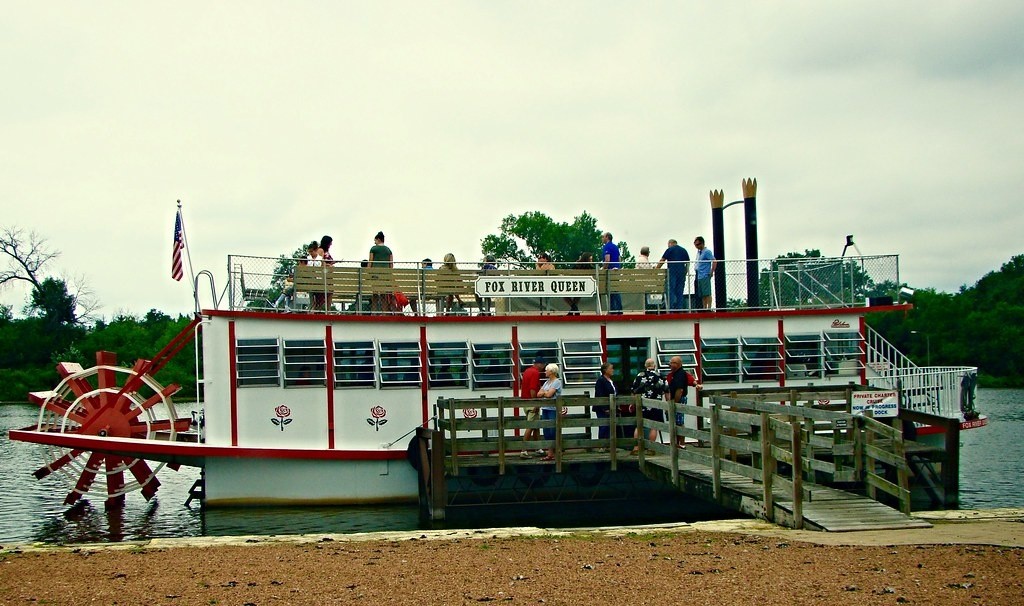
540, 455, 555, 461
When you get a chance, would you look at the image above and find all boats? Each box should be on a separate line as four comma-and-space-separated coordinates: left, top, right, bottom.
6, 234, 987, 514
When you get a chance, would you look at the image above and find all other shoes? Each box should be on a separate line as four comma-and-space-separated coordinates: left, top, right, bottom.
680, 444, 686, 449
630, 450, 639, 455
643, 449, 655, 456
598, 447, 605, 453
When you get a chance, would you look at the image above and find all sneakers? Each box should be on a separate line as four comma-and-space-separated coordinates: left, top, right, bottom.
520, 451, 531, 459
536, 449, 545, 454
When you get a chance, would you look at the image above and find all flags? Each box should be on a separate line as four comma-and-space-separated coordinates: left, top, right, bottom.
172, 208, 184, 282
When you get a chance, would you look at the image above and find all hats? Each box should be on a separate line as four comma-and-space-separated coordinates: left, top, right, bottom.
533, 357, 545, 364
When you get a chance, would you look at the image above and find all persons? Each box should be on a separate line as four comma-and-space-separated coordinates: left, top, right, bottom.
534, 257, 556, 271
666, 356, 703, 451
628, 357, 671, 456
520, 356, 548, 459
265, 235, 336, 315
537, 363, 561, 461
656, 239, 690, 314
353, 259, 370, 315
435, 253, 467, 316
473, 256, 500, 316
369, 231, 393, 316
694, 237, 717, 309
563, 253, 596, 316
602, 232, 624, 314
409, 259, 434, 316
591, 363, 623, 453
633, 246, 658, 315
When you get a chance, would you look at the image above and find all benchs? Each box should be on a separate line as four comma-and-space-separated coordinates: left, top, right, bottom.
290, 266, 670, 316
